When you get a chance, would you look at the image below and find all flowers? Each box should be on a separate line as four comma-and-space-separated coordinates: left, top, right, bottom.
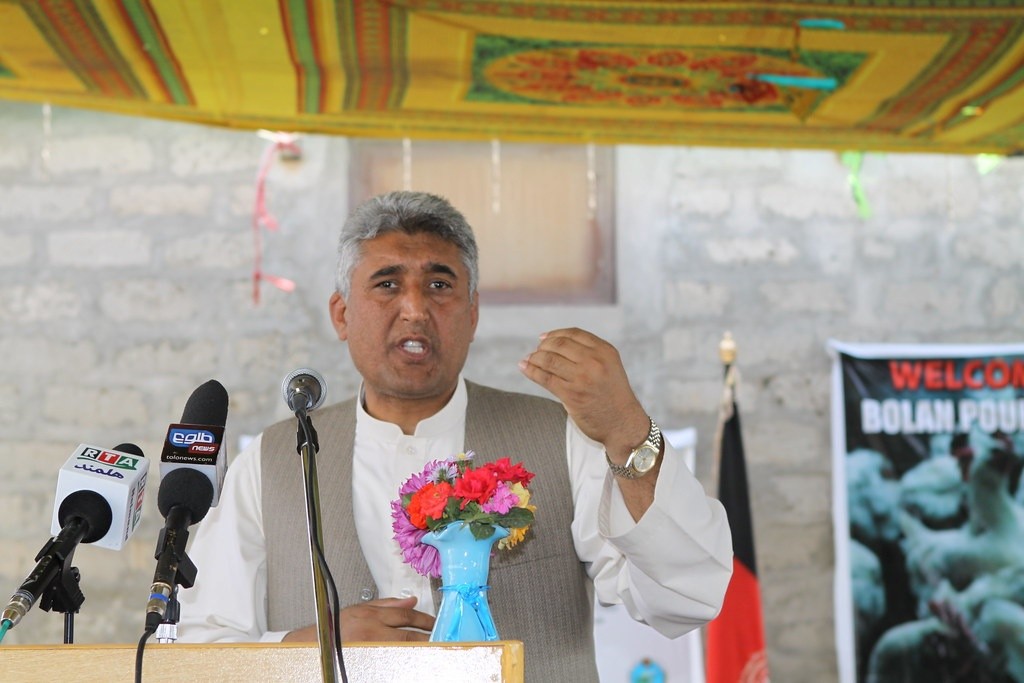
390, 450, 537, 580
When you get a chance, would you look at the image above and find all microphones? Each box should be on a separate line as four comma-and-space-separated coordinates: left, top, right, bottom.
1, 442, 151, 630
142, 379, 229, 634
280, 369, 327, 415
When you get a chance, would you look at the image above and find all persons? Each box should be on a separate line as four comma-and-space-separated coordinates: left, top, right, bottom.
175, 190, 735, 683
849, 426, 1024, 682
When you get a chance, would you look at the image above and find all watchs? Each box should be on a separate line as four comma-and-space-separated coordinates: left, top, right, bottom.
606, 418, 661, 479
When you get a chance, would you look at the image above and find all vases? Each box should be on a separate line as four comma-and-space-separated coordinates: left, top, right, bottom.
421, 521, 511, 642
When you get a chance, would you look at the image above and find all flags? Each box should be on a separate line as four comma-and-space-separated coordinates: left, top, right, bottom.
702, 366, 770, 683
